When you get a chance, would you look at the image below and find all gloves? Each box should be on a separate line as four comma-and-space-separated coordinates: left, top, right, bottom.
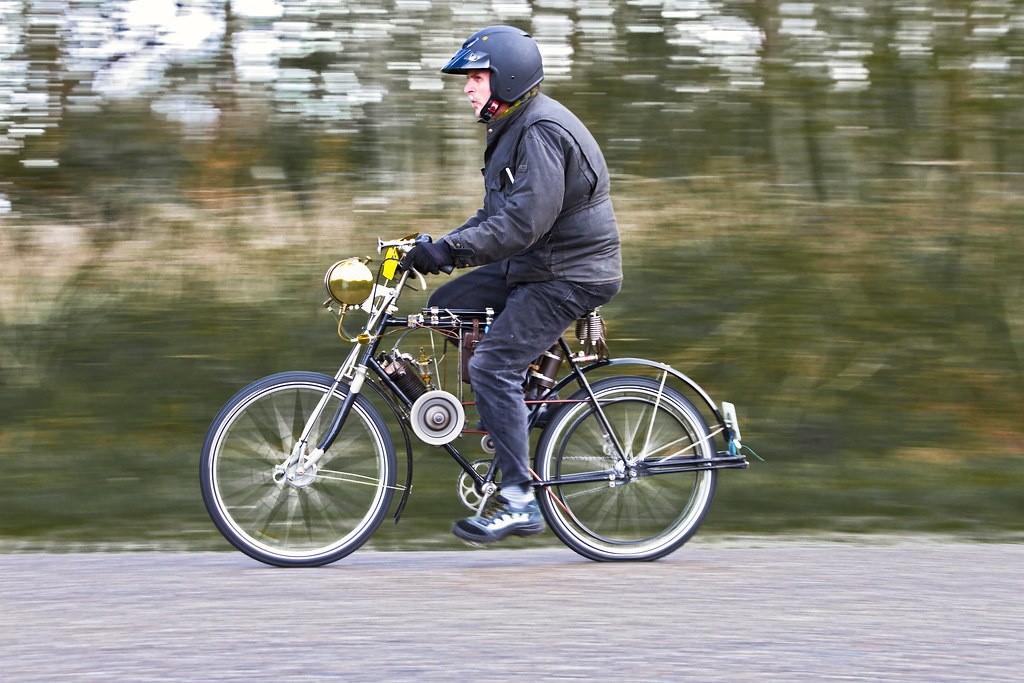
401, 238, 452, 279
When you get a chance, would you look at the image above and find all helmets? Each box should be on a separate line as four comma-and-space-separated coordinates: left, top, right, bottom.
441, 25, 543, 103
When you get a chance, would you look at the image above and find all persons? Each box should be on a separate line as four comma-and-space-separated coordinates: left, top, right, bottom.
400, 25, 623, 541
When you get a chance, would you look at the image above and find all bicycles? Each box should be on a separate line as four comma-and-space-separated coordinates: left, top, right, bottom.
199, 231, 765, 568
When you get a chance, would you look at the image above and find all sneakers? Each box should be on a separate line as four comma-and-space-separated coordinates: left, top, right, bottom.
452, 495, 545, 542
478, 394, 561, 428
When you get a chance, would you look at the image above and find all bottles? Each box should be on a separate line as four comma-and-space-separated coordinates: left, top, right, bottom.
374, 350, 426, 403
